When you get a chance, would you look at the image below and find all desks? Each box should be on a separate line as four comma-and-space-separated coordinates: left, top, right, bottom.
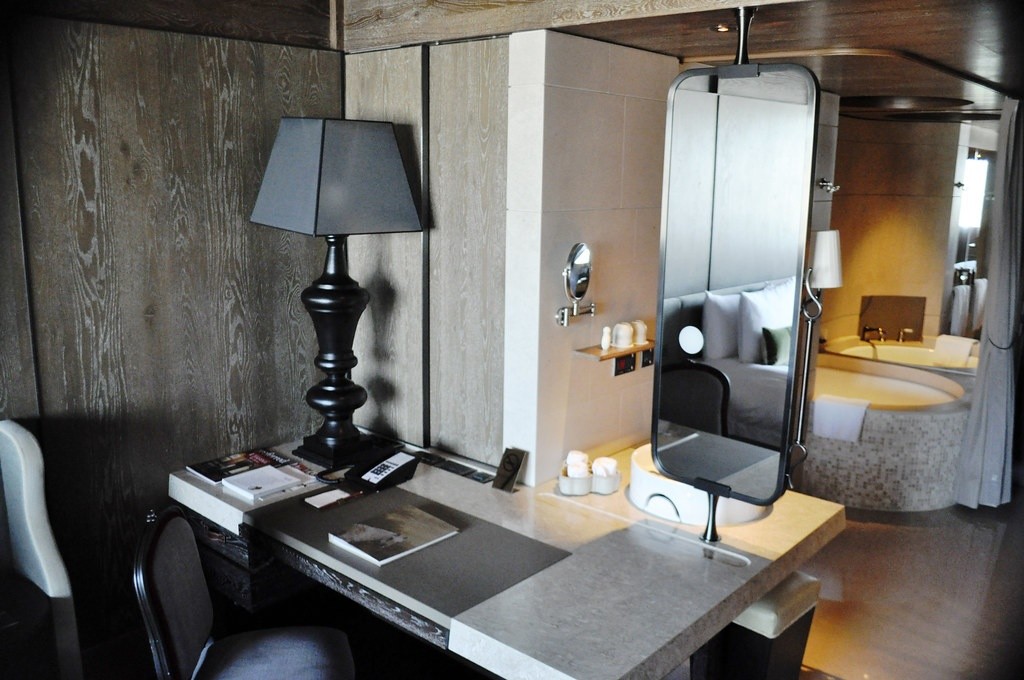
167, 425, 845, 680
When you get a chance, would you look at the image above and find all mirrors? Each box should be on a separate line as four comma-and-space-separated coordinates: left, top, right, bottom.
954, 147, 998, 286
649, 61, 820, 507
562, 242, 592, 303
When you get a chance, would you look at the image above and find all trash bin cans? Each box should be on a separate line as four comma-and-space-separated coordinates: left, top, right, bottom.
690, 570, 821, 680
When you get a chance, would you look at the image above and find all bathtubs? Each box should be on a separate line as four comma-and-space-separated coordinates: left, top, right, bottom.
793, 351, 977, 510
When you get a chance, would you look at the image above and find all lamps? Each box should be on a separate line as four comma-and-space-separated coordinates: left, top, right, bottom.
251, 114, 424, 467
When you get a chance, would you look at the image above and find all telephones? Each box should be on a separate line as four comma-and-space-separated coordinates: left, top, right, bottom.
343, 446, 422, 493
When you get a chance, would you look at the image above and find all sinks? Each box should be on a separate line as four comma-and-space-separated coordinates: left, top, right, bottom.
630, 444, 767, 526
820, 334, 980, 368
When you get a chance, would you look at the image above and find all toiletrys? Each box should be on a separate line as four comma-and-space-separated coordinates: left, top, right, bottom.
818, 328, 826, 351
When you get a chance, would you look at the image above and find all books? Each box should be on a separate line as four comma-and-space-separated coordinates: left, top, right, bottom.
259, 462, 322, 500
185, 447, 298, 484
328, 504, 459, 567
221, 465, 301, 500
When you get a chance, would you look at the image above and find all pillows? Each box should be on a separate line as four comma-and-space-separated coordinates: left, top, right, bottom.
739, 276, 797, 363
761, 327, 792, 365
702, 290, 740, 361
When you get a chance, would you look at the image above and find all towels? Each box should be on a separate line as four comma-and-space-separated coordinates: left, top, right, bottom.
931, 334, 981, 367
951, 284, 971, 337
565, 450, 619, 477
971, 278, 988, 334
813, 394, 871, 444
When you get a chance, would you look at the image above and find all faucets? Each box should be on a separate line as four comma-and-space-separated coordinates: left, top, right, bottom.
860, 326, 885, 342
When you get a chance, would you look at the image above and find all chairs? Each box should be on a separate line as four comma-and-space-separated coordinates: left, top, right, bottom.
0, 420, 82, 680
132, 506, 354, 680
661, 359, 731, 438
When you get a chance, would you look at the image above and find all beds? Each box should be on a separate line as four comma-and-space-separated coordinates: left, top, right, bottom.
664, 278, 797, 449
